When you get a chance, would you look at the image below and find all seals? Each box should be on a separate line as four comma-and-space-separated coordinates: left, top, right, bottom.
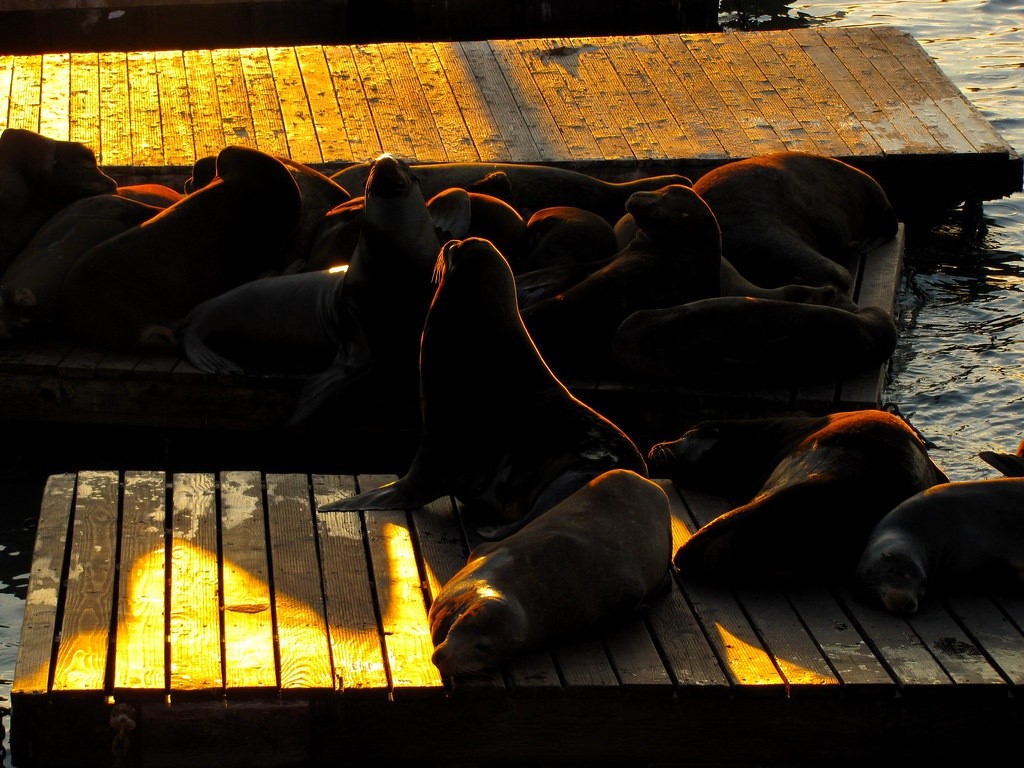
0, 128, 901, 541
649, 409, 1024, 615
428, 468, 674, 676
